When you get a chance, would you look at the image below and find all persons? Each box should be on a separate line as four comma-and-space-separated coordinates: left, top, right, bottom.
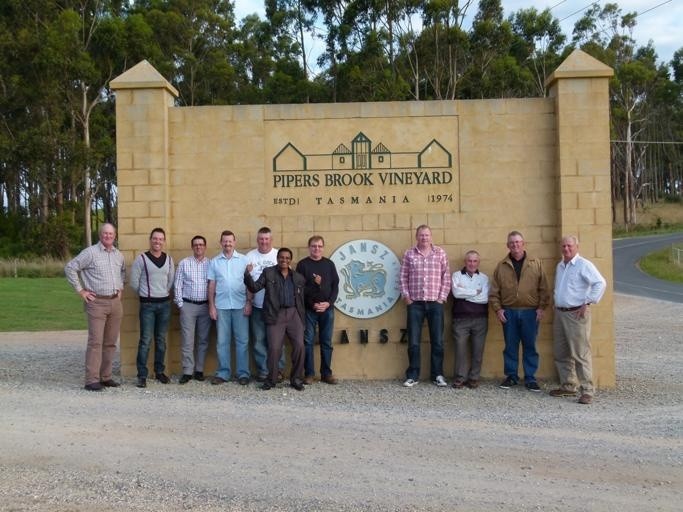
172, 226, 338, 391
448, 250, 489, 388
129, 227, 173, 387
488, 231, 550, 391
399, 224, 452, 387
549, 235, 607, 403
63, 223, 127, 390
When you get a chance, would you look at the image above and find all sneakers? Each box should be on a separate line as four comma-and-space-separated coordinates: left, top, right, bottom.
498, 377, 518, 389
578, 393, 593, 404
256, 376, 283, 382
432, 375, 447, 387
401, 378, 420, 387
524, 382, 541, 393
304, 375, 316, 385
549, 387, 577, 397
320, 375, 338, 384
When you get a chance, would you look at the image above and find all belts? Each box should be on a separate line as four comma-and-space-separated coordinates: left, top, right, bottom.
555, 303, 590, 312
90, 292, 118, 299
182, 297, 207, 306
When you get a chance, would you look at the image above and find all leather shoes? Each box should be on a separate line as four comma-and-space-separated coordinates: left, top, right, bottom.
238, 376, 249, 385
155, 373, 170, 384
452, 380, 464, 388
210, 376, 225, 385
103, 380, 120, 388
261, 382, 275, 389
466, 379, 478, 388
290, 381, 304, 391
193, 372, 204, 381
137, 377, 146, 388
83, 383, 101, 391
179, 374, 191, 384
